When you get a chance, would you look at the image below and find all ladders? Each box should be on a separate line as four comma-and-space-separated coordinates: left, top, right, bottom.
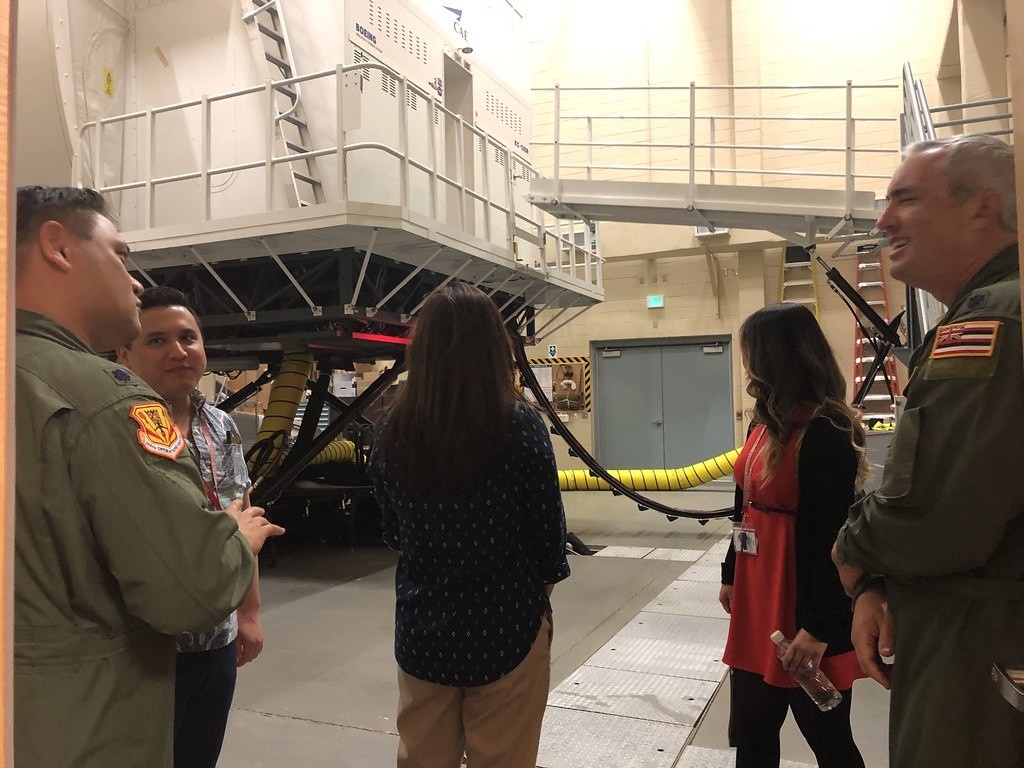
780, 247, 822, 328
853, 242, 901, 424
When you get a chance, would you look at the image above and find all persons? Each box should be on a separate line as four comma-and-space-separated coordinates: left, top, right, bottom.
833, 134, 1024, 768
719, 302, 870, 768
115, 285, 264, 768
364, 281, 570, 768
16, 186, 286, 768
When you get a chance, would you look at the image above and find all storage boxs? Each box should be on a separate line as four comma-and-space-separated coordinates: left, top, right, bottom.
228, 363, 406, 445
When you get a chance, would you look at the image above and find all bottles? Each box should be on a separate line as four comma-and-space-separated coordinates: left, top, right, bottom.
770, 629, 842, 713
